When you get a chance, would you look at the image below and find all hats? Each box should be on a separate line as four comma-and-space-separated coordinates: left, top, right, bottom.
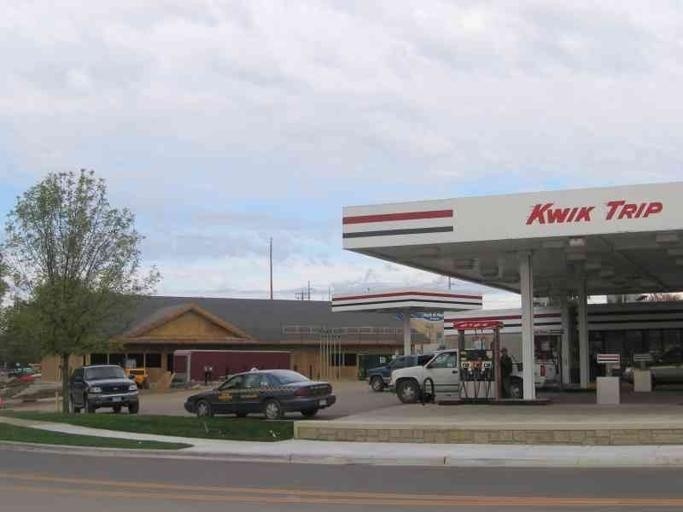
501, 348, 507, 352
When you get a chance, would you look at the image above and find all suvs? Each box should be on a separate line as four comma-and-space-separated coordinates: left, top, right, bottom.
65, 363, 143, 417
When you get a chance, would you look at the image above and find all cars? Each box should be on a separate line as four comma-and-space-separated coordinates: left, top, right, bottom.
0, 367, 43, 381
622, 344, 682, 390
183, 367, 336, 422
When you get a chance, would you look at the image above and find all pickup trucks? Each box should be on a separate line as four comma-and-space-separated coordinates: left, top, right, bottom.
391, 347, 547, 404
365, 352, 433, 393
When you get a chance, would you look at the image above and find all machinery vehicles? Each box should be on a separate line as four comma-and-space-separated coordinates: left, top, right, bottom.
126, 367, 151, 390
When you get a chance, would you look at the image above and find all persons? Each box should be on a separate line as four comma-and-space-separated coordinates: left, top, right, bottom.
499, 346, 513, 398
